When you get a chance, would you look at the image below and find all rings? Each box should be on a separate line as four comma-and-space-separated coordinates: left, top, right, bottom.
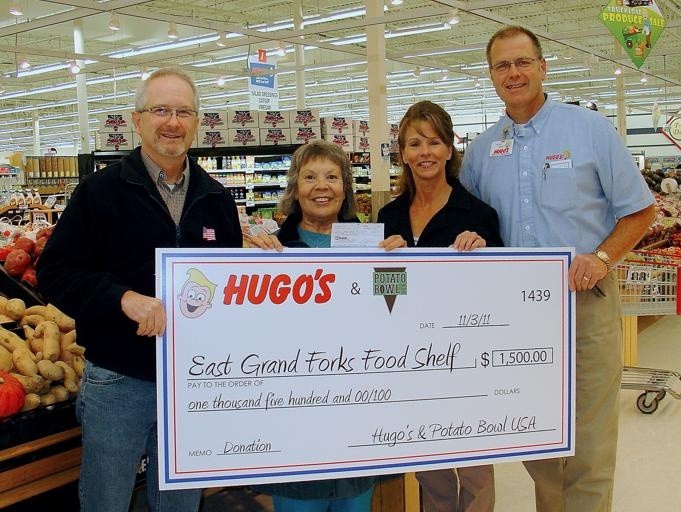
583, 272, 593, 282
262, 236, 271, 241
461, 237, 468, 243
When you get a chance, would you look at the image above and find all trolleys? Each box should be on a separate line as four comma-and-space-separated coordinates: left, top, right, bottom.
615, 250, 681, 414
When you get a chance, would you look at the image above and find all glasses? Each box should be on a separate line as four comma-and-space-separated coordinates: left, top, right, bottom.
489, 57, 539, 71
138, 106, 197, 117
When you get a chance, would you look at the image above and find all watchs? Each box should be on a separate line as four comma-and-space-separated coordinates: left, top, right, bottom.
592, 248, 614, 273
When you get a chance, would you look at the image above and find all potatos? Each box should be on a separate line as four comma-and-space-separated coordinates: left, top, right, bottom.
4, 227, 54, 286
0, 294, 88, 413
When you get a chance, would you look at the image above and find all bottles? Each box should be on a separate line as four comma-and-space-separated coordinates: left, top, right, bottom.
196, 156, 246, 213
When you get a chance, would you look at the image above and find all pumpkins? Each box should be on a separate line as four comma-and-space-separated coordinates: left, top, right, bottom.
0, 372, 26, 419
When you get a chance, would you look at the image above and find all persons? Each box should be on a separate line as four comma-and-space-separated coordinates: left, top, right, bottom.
245, 138, 408, 512
37, 67, 245, 510
50, 149, 58, 157
376, 99, 507, 512
458, 25, 658, 510
